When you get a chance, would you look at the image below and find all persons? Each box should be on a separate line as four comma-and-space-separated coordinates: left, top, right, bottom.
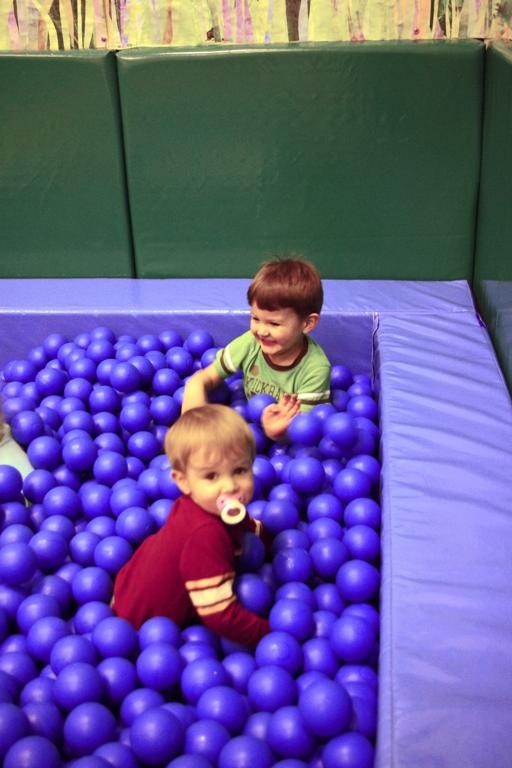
110, 403, 271, 650
180, 256, 333, 448
0, 414, 34, 479
